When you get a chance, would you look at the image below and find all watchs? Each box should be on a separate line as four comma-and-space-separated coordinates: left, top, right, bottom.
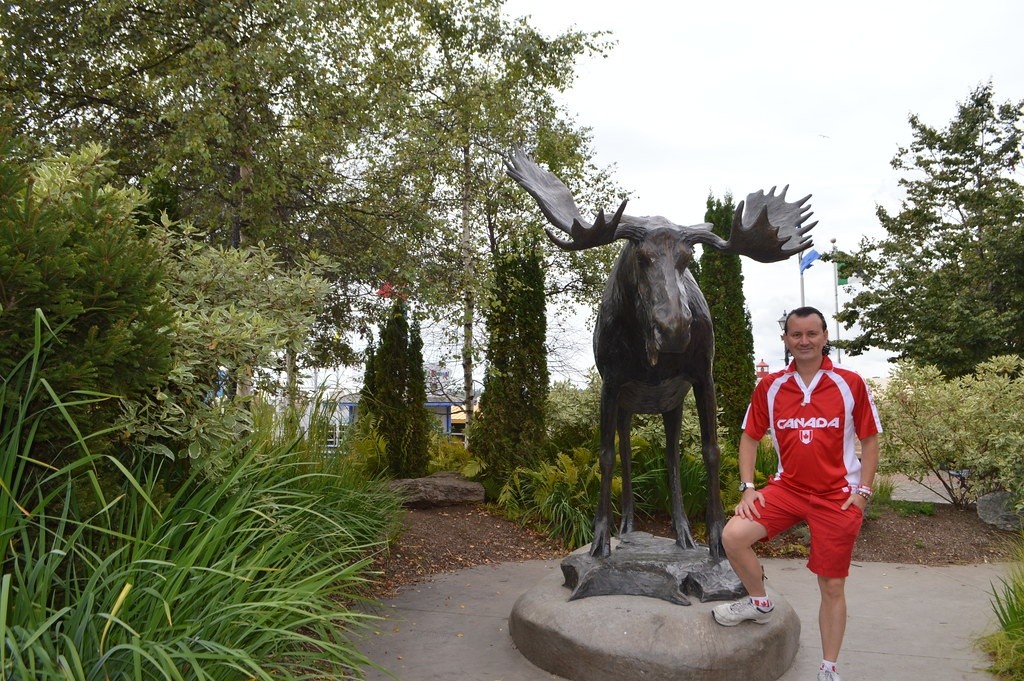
738, 482, 755, 492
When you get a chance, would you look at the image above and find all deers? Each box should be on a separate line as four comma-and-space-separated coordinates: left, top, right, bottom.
499, 142, 820, 558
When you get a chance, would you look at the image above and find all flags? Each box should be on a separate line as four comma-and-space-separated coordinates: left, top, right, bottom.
800, 249, 822, 274
838, 251, 868, 285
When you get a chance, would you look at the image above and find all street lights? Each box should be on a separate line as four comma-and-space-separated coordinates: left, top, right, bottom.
777, 309, 788, 367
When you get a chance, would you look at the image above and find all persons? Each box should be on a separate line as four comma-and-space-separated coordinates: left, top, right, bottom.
711, 307, 883, 681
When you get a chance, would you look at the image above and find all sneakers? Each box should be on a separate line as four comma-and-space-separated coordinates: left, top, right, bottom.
712, 595, 775, 626
817, 668, 840, 681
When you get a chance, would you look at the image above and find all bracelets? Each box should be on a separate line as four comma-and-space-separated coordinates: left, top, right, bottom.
856, 485, 874, 504
